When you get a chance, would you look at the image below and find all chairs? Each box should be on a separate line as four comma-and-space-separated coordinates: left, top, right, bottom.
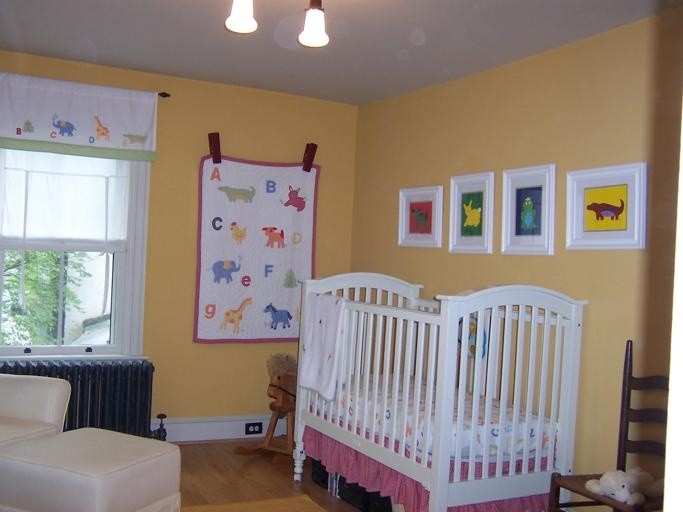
1, 373, 71, 442
549, 341, 669, 511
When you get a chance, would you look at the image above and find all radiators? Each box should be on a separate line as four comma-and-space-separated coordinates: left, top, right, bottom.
1, 358, 167, 441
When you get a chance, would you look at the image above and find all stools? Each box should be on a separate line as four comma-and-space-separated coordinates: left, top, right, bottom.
1, 426, 182, 512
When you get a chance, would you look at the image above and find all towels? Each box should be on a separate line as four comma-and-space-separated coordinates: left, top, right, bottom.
298, 292, 350, 401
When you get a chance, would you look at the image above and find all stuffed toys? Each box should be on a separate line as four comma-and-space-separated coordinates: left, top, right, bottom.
585, 466, 664, 505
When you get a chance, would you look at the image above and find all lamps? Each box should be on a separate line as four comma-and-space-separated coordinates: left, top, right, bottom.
296, 1, 331, 48
224, 0, 257, 34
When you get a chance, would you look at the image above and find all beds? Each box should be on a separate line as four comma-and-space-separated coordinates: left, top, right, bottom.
291, 271, 589, 512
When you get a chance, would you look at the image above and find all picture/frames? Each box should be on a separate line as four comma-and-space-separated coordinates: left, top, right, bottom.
397, 185, 443, 248
565, 161, 647, 251
500, 164, 556, 256
448, 172, 495, 255
